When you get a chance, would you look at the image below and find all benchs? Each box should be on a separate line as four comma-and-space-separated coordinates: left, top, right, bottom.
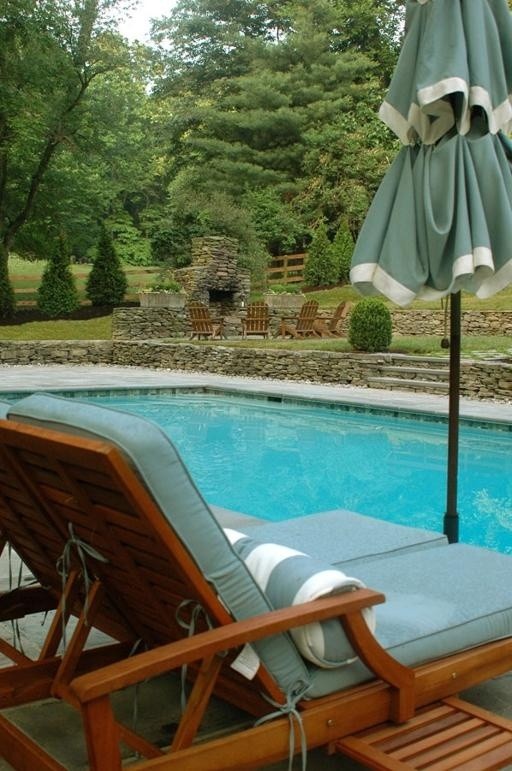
0, 391, 512, 770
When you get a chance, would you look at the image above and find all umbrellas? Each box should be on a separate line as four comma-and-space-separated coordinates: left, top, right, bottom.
341, 2, 512, 545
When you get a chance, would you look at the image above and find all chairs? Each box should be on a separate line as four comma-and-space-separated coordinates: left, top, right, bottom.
188, 300, 351, 341
